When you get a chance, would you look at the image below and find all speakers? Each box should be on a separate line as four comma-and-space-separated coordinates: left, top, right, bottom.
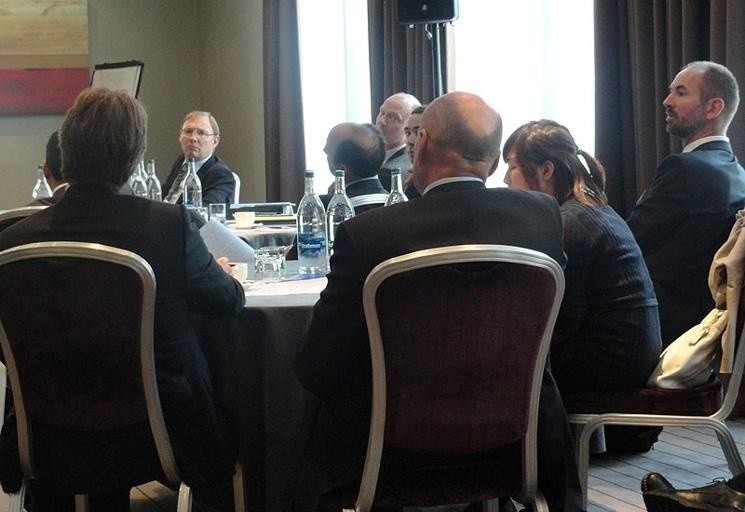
397, 0, 458, 24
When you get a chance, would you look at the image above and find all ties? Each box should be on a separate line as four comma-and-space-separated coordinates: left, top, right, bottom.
167, 162, 188, 202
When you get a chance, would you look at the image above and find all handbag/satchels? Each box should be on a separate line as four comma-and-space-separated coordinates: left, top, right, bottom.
647, 307, 729, 389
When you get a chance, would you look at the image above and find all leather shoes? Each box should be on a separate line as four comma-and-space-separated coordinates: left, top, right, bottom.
641, 472, 745, 512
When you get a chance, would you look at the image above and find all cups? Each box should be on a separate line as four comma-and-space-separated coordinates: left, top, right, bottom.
229, 262, 248, 285
209, 203, 255, 230
254, 245, 287, 283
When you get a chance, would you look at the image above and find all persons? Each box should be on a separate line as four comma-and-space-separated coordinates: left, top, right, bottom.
605, 59, 745, 458
290, 85, 661, 511
1, 85, 245, 512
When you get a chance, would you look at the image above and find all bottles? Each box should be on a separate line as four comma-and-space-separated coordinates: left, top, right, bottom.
128, 157, 206, 209
295, 167, 410, 280
32, 164, 53, 199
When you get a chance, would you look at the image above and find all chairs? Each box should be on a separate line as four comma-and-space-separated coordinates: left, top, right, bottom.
317, 241, 567, 512
4, 205, 51, 230
345, 194, 391, 216
0, 239, 246, 512
231, 171, 241, 205
565, 276, 745, 512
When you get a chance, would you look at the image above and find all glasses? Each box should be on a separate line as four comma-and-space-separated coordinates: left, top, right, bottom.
178, 128, 215, 139
